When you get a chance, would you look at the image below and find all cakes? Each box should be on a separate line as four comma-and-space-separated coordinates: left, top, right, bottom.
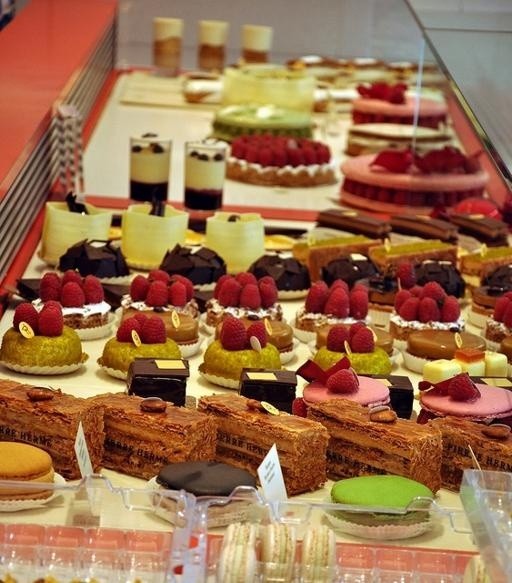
353, 83, 445, 123
225, 66, 314, 109
210, 104, 315, 140
347, 124, 451, 157
227, 134, 335, 185
340, 145, 486, 213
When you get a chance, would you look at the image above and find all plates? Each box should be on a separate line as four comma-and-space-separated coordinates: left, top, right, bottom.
97, 356, 127, 380
0, 472, 68, 513
197, 362, 241, 390
98, 272, 311, 301
321, 495, 442, 542
280, 338, 301, 366
73, 312, 118, 341
1, 350, 90, 375
178, 333, 205, 357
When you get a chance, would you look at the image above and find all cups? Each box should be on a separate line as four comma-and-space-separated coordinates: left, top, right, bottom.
240, 24, 270, 66
40, 200, 112, 264
205, 211, 265, 273
198, 19, 226, 76
121, 205, 189, 269
152, 16, 182, 70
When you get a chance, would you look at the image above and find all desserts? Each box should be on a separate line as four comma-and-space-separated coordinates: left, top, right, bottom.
0, 198, 512, 390
197, 21, 229, 68
0, 358, 512, 582
154, 19, 182, 67
243, 26, 273, 62
